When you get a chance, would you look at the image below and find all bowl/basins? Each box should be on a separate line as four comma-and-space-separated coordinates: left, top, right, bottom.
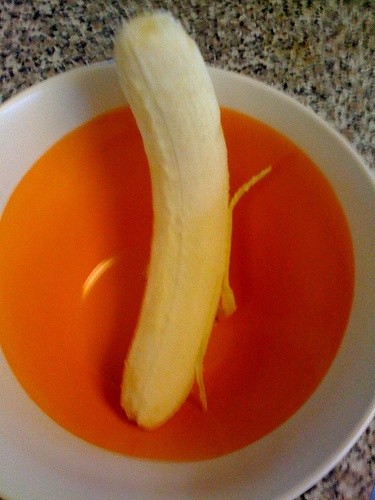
0, 59, 375, 499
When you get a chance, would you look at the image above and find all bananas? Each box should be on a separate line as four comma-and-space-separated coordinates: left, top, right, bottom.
114, 9, 231, 431
